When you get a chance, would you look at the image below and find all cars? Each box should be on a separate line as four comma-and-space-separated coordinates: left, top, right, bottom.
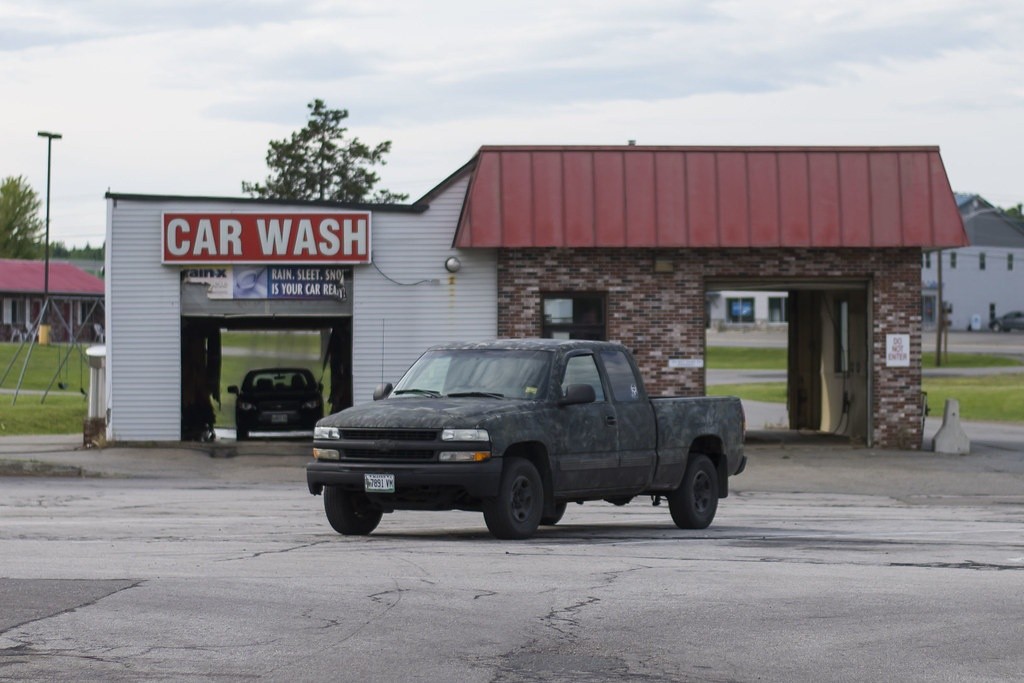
227, 367, 324, 440
988, 311, 1024, 332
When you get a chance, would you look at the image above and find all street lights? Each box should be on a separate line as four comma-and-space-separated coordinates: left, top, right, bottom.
38, 133, 63, 297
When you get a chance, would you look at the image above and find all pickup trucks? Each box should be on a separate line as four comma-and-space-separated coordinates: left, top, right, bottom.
306, 337, 748, 540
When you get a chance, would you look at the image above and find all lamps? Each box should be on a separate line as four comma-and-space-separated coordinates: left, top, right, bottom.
654, 255, 675, 273
445, 257, 460, 273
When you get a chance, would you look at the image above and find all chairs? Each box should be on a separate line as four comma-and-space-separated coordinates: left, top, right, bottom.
257, 378, 273, 387
93, 323, 105, 341
10, 323, 39, 342
